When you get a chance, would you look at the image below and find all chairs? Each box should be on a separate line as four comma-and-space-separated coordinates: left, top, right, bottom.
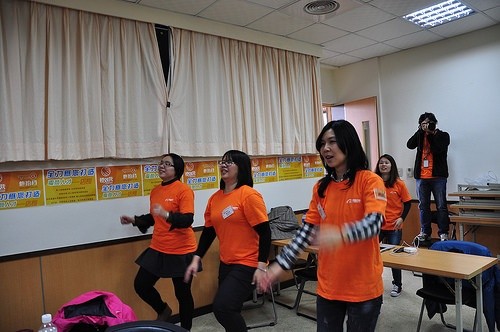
295, 249, 318, 323
411, 210, 458, 277
416, 239, 496, 332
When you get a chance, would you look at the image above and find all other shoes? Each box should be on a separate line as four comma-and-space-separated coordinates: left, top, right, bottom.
156, 303, 172, 320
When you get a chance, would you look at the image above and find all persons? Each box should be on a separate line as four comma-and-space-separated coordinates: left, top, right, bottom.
407, 112, 450, 243
120, 153, 202, 332
184, 150, 273, 332
265, 120, 387, 332
373, 154, 412, 298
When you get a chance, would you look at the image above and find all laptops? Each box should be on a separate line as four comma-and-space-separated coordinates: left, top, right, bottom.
379, 244, 395, 252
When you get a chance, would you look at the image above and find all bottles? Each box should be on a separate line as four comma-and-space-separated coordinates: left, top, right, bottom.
38, 313, 58, 332
302, 212, 306, 225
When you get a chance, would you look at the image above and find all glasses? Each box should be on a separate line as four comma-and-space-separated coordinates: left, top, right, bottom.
218, 161, 235, 166
158, 162, 174, 168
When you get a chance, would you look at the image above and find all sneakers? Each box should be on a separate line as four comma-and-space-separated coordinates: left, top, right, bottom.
441, 234, 448, 241
415, 233, 431, 240
391, 284, 401, 296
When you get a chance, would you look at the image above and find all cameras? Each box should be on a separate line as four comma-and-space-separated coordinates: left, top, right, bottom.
422, 121, 436, 131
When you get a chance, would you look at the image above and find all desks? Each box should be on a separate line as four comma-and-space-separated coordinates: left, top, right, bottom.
270, 239, 498, 332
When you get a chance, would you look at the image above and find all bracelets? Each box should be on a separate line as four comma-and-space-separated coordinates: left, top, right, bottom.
166, 212, 169, 218
257, 267, 267, 273
